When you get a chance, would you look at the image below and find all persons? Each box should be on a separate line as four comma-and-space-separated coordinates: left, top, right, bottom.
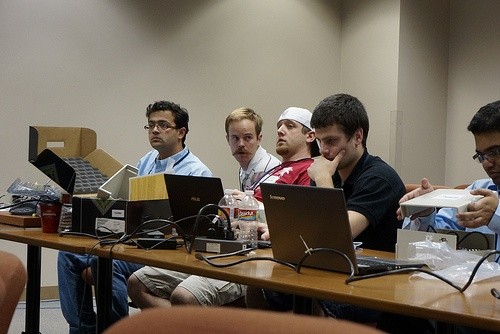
127, 107, 321, 310
229, 95, 406, 322
57, 101, 216, 334
0, 251, 27, 334
224, 108, 281, 191
396, 100, 500, 334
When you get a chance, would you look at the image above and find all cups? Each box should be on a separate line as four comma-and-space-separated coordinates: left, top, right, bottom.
38, 201, 64, 233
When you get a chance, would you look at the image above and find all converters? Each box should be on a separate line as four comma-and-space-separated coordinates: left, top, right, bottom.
137, 237, 178, 249
192, 237, 242, 252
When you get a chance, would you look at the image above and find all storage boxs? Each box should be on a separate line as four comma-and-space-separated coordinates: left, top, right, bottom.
26, 123, 175, 239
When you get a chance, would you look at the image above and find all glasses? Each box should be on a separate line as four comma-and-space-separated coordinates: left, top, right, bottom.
472, 147, 500, 164
144, 122, 181, 130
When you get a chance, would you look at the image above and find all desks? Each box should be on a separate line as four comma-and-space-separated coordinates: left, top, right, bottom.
0, 208, 111, 334
111, 240, 500, 332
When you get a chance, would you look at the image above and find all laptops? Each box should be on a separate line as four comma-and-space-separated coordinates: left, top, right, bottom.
259, 182, 430, 275
163, 174, 223, 236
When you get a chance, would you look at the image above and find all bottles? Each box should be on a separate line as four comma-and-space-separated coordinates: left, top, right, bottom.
238, 189, 259, 256
218, 189, 239, 239
58, 190, 72, 233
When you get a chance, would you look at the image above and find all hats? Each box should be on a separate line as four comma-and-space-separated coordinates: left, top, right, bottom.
277, 107, 321, 158
